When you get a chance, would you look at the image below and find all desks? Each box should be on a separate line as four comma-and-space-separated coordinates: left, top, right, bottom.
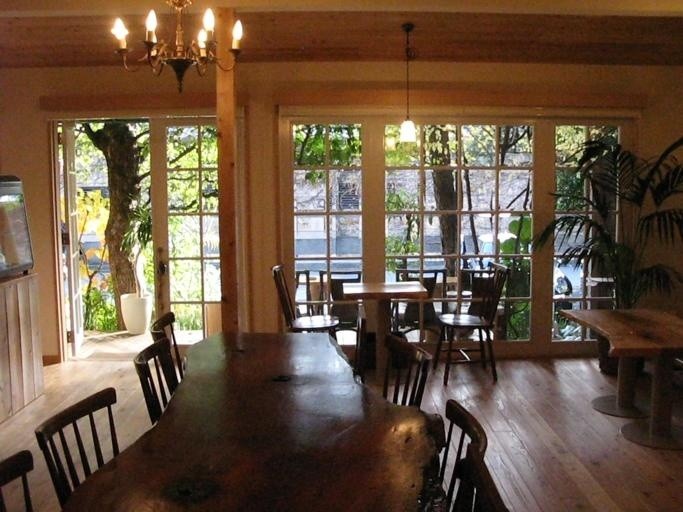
559, 308, 683, 451
66, 332, 447, 512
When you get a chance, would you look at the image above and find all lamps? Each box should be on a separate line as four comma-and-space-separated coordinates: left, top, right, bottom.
399, 30, 418, 144
110, 2, 244, 95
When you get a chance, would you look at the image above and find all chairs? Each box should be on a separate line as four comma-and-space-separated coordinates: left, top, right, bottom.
272, 261, 510, 385
134, 339, 177, 427
151, 312, 184, 395
34, 387, 118, 510
0, 451, 35, 512
439, 400, 486, 504
444, 444, 509, 512
384, 332, 433, 408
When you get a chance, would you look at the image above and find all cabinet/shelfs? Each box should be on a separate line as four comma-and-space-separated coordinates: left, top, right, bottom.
0, 272, 45, 425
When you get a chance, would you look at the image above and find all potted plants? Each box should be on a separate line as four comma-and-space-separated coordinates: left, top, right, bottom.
530, 135, 683, 376
119, 205, 153, 335
